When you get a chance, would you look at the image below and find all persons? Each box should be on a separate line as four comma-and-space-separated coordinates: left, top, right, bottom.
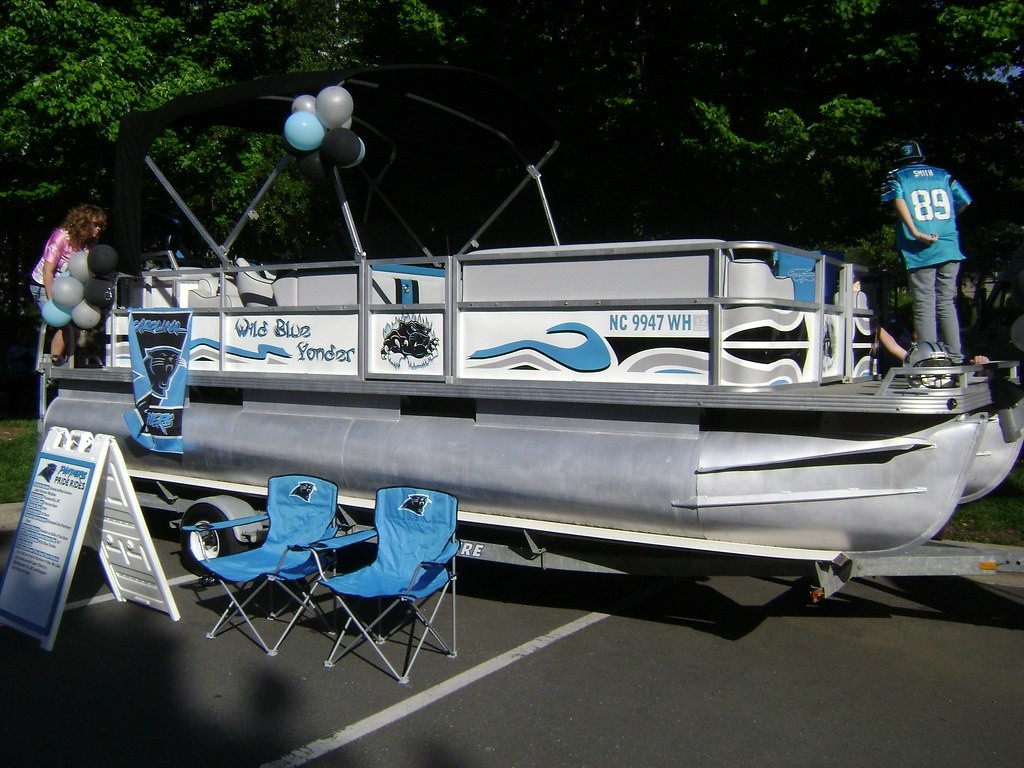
880, 326, 990, 364
879, 140, 974, 354
30, 204, 107, 361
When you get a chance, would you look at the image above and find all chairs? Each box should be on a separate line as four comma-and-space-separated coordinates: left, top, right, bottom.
180, 474, 356, 656
295, 485, 461, 685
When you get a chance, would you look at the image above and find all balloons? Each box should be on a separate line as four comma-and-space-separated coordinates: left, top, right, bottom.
42, 244, 118, 328
283, 85, 366, 179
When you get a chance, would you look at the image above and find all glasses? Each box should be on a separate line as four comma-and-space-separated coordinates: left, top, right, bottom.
92, 222, 99, 227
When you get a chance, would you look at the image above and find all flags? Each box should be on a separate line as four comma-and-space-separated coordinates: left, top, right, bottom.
123, 307, 193, 454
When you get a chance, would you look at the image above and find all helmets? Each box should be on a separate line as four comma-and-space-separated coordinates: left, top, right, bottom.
903, 341, 956, 389
894, 140, 926, 162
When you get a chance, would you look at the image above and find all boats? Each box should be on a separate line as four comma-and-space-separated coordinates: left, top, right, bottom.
34, 65, 1024, 605
